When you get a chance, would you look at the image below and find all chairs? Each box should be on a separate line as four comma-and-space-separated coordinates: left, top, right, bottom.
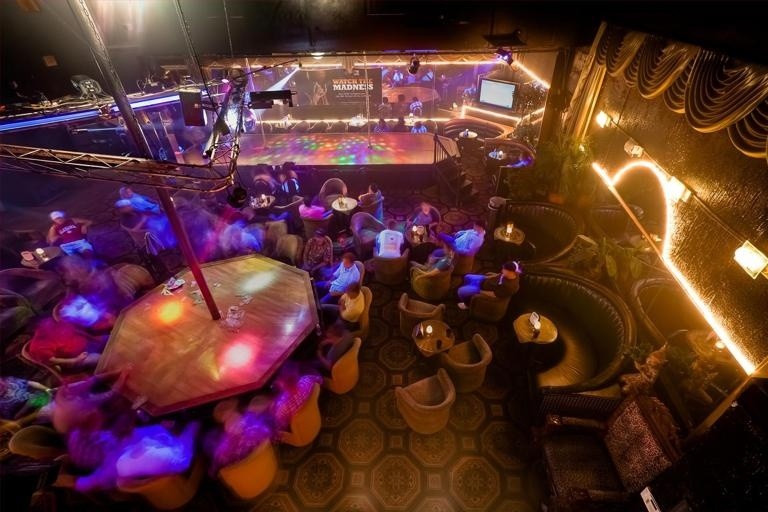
0, 72, 687, 512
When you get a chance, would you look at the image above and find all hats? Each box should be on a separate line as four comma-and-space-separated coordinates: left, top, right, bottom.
50, 212, 63, 221
229, 188, 247, 208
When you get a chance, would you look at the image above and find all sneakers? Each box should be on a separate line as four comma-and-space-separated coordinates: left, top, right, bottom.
458, 303, 469, 310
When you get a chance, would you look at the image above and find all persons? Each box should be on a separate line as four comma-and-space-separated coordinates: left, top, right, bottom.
421, 67, 434, 81
375, 219, 404, 260
409, 121, 427, 134
410, 201, 438, 238
392, 92, 409, 117
372, 119, 392, 132
392, 116, 411, 134
505, 150, 529, 169
1, 160, 366, 493
455, 260, 523, 312
426, 219, 486, 262
391, 70, 404, 83
373, 97, 392, 118
408, 96, 425, 115
409, 242, 457, 272
358, 181, 381, 204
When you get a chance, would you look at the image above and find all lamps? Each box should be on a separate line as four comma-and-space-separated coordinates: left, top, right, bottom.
408, 57, 420, 75
733, 240, 768, 280
495, 48, 513, 66
